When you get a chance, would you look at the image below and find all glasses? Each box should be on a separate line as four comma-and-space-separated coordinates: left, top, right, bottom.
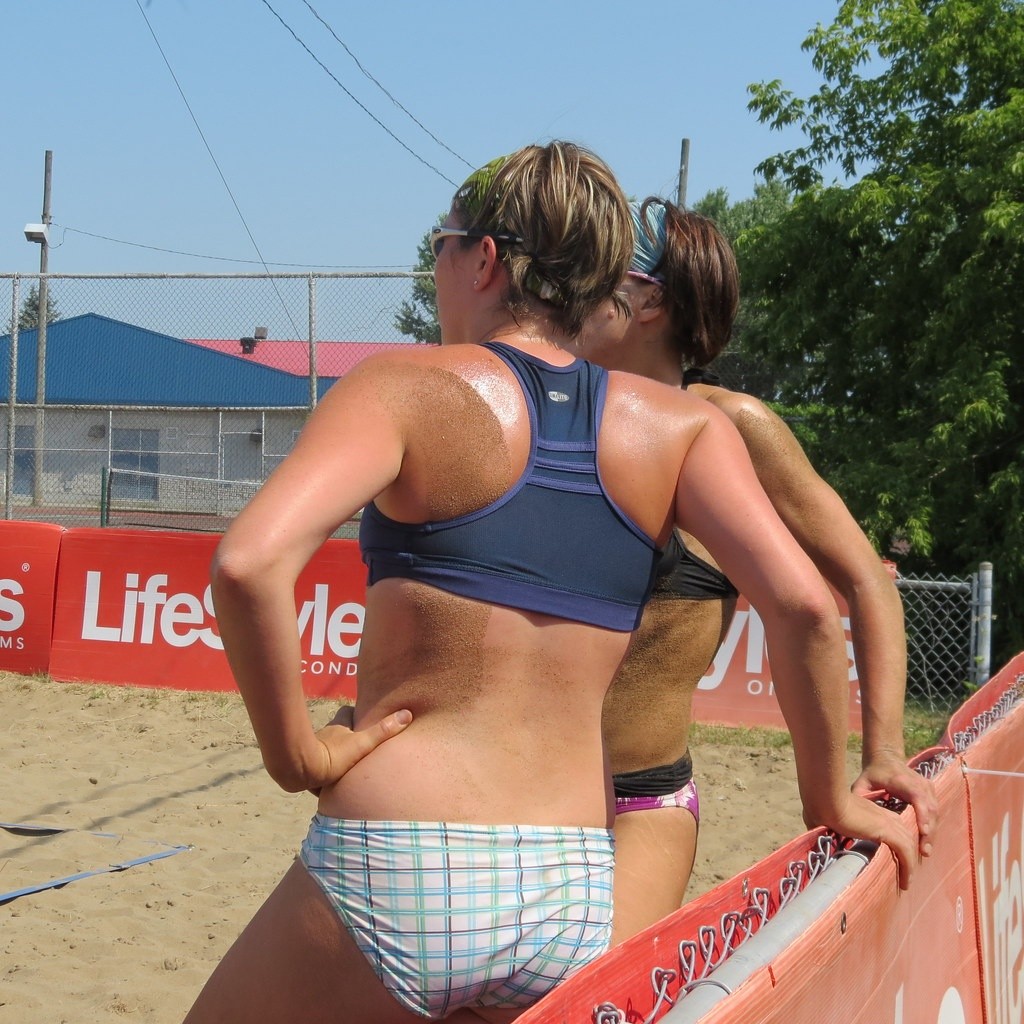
430, 225, 519, 261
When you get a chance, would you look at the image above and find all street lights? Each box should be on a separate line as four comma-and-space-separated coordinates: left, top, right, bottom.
24, 223, 49, 508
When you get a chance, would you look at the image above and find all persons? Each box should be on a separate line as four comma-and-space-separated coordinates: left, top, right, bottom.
180, 140, 938, 1024
